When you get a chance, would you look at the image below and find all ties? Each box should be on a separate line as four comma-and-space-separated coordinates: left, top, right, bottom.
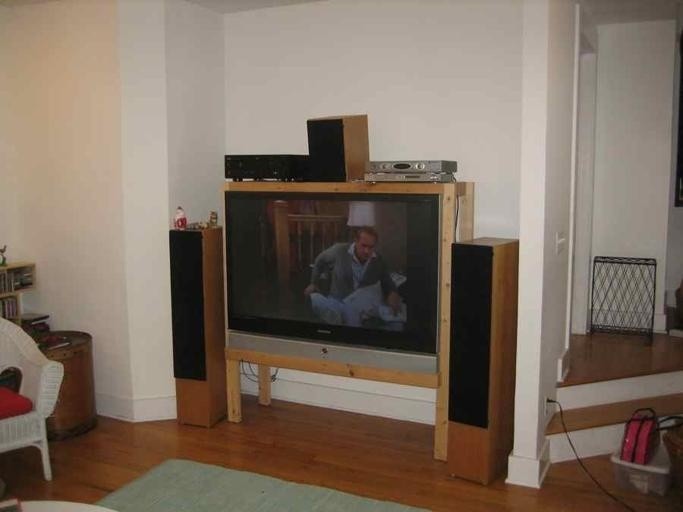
220, 189, 444, 375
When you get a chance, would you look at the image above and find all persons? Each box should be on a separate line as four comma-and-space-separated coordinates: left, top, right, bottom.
300, 226, 402, 315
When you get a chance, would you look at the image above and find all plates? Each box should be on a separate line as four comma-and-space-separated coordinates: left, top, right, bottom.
0, 259, 36, 328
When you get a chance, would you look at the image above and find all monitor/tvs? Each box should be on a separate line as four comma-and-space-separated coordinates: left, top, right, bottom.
0, 270, 17, 319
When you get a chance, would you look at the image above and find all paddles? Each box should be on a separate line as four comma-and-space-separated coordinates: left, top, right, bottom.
92, 454, 432, 511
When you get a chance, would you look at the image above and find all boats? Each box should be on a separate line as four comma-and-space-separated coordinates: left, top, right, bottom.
305, 114, 371, 182
167, 226, 227, 429
444, 236, 522, 489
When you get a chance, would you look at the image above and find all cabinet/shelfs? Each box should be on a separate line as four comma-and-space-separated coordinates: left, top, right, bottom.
173, 205, 187, 231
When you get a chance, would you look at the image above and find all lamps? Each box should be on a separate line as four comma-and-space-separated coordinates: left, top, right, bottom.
0, 318, 64, 481
